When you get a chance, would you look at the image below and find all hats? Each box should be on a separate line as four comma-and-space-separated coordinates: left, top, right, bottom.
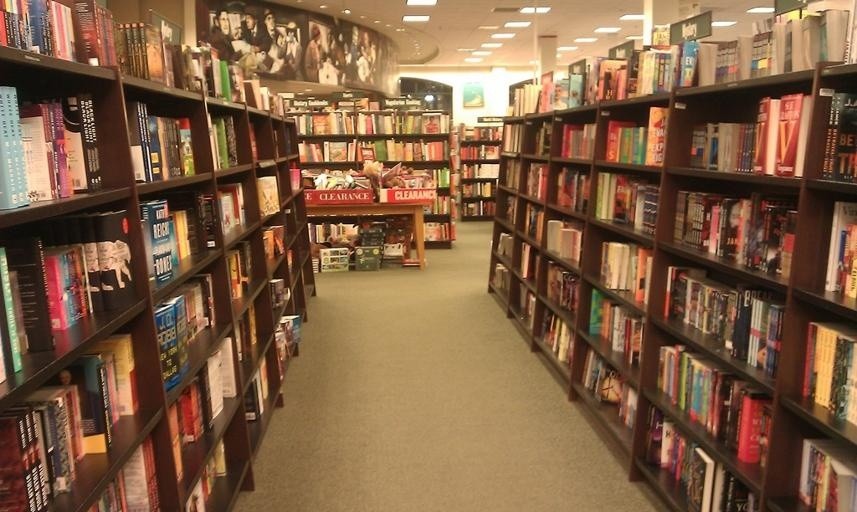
263, 8, 275, 19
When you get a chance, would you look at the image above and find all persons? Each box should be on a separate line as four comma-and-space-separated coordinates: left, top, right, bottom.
209, 8, 383, 88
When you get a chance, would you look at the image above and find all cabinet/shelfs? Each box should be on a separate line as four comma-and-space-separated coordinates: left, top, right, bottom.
482, 66, 855, 511
291, 107, 499, 273
1, 41, 316, 510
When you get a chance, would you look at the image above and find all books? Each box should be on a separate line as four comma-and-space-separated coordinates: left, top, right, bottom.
1, 1, 307, 512
489, 10, 855, 511
455, 122, 502, 216
288, 98, 459, 269
